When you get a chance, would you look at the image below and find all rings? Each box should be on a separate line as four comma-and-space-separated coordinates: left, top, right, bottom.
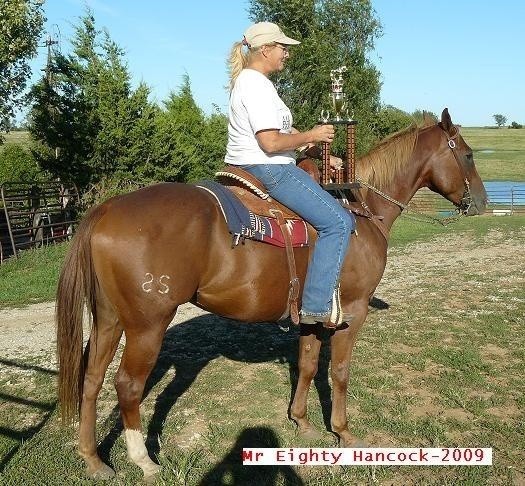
328, 132, 332, 138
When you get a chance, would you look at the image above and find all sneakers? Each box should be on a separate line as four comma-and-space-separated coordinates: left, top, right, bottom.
300, 314, 352, 325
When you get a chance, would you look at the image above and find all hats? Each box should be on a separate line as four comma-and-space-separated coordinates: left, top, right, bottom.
244, 23, 301, 49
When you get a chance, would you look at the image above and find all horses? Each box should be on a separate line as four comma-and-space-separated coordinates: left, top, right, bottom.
55, 107, 488, 479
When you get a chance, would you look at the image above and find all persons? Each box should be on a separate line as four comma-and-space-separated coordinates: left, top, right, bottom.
221, 21, 354, 329
